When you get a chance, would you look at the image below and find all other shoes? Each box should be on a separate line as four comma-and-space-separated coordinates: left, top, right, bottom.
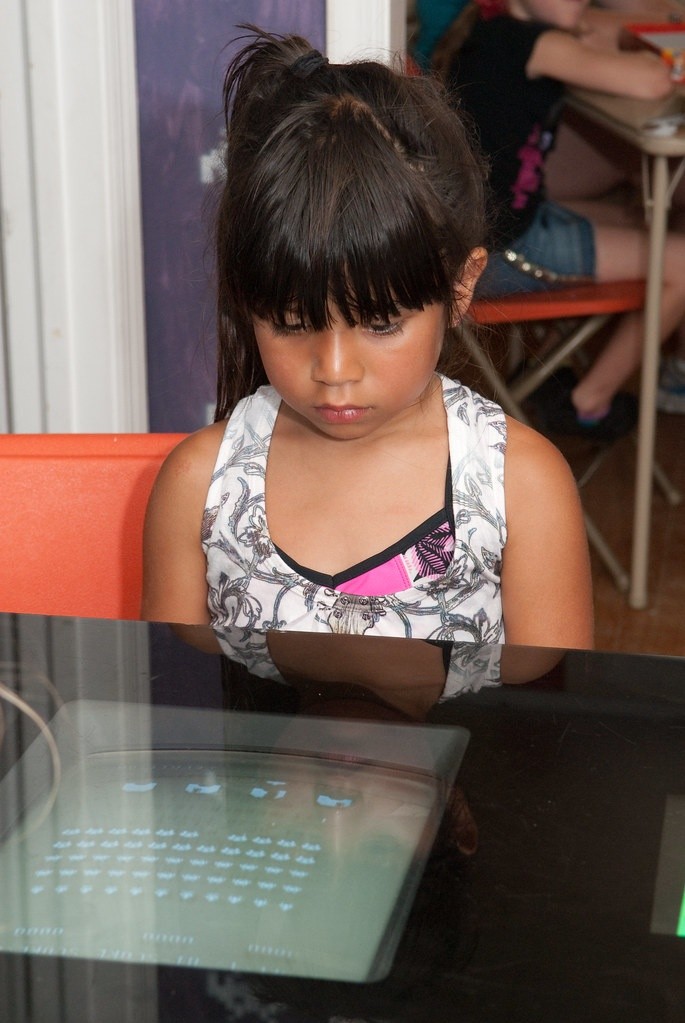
541, 384, 640, 439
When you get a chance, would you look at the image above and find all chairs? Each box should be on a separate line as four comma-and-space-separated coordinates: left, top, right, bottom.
450, 281, 683, 596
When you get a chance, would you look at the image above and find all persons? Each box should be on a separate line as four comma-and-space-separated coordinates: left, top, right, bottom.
171, 624, 564, 1018
139, 19, 596, 649
418, 0, 685, 444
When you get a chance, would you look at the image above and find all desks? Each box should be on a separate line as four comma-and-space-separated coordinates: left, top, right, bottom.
561, 86, 685, 609
0, 610, 685, 1023
